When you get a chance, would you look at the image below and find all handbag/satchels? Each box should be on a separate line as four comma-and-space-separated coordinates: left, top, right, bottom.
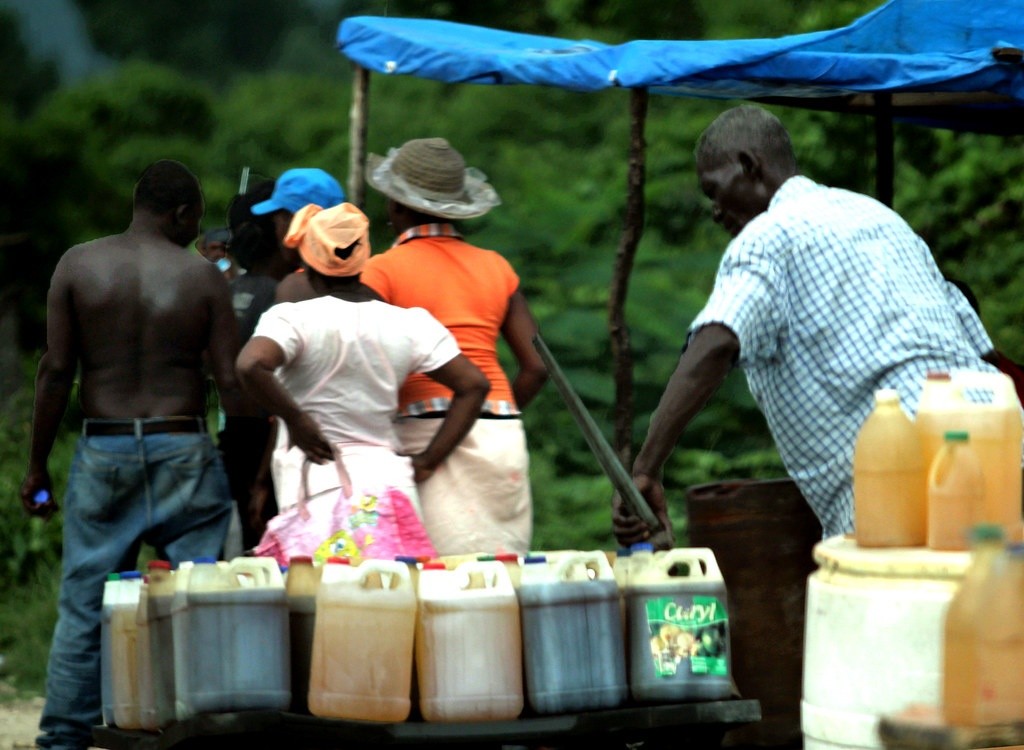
243, 442, 440, 563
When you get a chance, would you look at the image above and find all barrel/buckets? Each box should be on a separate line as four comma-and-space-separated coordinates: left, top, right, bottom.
682, 475, 827, 748
798, 532, 975, 750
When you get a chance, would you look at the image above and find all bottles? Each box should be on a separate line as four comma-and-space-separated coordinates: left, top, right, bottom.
916, 368, 1023, 549
939, 523, 1024, 732
92, 541, 737, 734
927, 428, 994, 551
852, 387, 931, 550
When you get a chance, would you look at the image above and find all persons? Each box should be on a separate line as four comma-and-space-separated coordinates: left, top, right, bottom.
355, 135, 552, 559
611, 101, 1024, 750
236, 203, 495, 566
17, 159, 249, 750
245, 167, 351, 556
215, 179, 293, 550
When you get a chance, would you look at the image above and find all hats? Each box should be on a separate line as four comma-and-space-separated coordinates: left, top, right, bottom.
250, 168, 346, 217
363, 137, 502, 219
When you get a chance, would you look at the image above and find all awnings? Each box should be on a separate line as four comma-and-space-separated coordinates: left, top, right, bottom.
337, 0, 1024, 233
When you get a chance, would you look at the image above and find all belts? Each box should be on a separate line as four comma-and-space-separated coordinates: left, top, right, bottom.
84, 418, 208, 435
